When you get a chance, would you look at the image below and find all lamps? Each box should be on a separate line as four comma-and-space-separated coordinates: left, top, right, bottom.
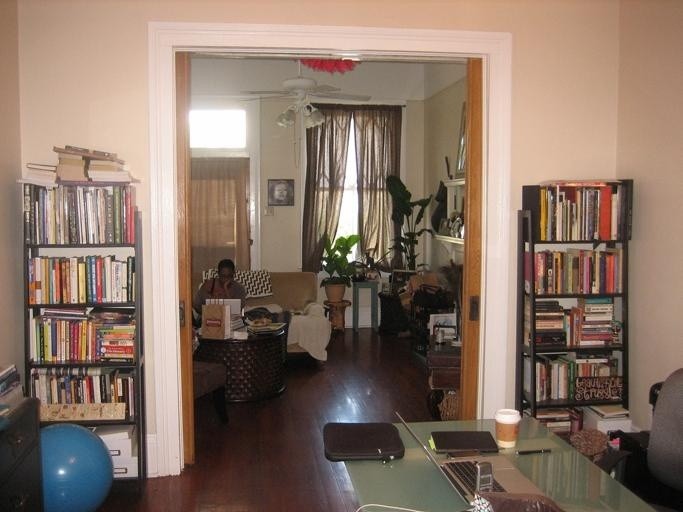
276, 59, 326, 133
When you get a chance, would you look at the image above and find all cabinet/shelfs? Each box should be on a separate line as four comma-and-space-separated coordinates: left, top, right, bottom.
23, 179, 147, 501
515, 210, 631, 439
1, 391, 45, 511
434, 178, 467, 270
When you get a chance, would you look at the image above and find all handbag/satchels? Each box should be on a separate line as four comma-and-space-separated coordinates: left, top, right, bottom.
202, 294, 231, 340
324, 423, 404, 464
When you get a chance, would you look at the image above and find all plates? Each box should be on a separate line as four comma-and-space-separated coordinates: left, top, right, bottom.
444, 336, 456, 339
249, 322, 285, 330
450, 344, 463, 346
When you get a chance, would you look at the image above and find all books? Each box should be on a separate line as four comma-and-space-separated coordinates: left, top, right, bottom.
23, 183, 136, 245
1, 363, 26, 415
30, 367, 134, 421
16, 145, 132, 185
28, 255, 136, 305
29, 308, 136, 362
520, 179, 629, 436
427, 430, 498, 456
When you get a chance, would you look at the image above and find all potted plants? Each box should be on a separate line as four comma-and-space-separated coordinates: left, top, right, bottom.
350, 241, 392, 280
320, 230, 365, 301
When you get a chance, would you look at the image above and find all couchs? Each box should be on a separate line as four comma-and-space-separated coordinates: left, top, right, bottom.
200, 270, 322, 368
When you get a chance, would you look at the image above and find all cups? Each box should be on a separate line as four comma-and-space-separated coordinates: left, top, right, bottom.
436, 330, 445, 343
495, 408, 520, 450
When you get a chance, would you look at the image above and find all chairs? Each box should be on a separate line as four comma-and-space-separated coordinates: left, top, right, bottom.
592, 366, 682, 512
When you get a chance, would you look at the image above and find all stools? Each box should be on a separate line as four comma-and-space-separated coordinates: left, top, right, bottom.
192, 358, 229, 462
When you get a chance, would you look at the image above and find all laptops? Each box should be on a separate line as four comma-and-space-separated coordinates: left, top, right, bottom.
393, 411, 547, 507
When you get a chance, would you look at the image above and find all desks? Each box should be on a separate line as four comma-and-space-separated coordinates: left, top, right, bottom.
426, 345, 461, 391
199, 320, 289, 406
343, 413, 657, 512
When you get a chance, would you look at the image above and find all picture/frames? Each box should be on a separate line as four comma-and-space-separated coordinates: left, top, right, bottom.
267, 178, 295, 207
456, 99, 467, 178
430, 314, 457, 340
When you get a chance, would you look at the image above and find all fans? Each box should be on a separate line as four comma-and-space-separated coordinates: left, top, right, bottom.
238, 57, 371, 104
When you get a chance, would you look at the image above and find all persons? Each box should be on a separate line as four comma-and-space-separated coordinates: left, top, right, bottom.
191, 260, 247, 319
269, 180, 289, 205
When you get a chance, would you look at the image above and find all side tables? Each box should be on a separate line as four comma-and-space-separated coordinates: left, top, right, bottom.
351, 281, 379, 334
378, 292, 411, 334
323, 300, 350, 333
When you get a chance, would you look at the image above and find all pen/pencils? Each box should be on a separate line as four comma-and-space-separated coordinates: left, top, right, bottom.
519, 448, 551, 454
